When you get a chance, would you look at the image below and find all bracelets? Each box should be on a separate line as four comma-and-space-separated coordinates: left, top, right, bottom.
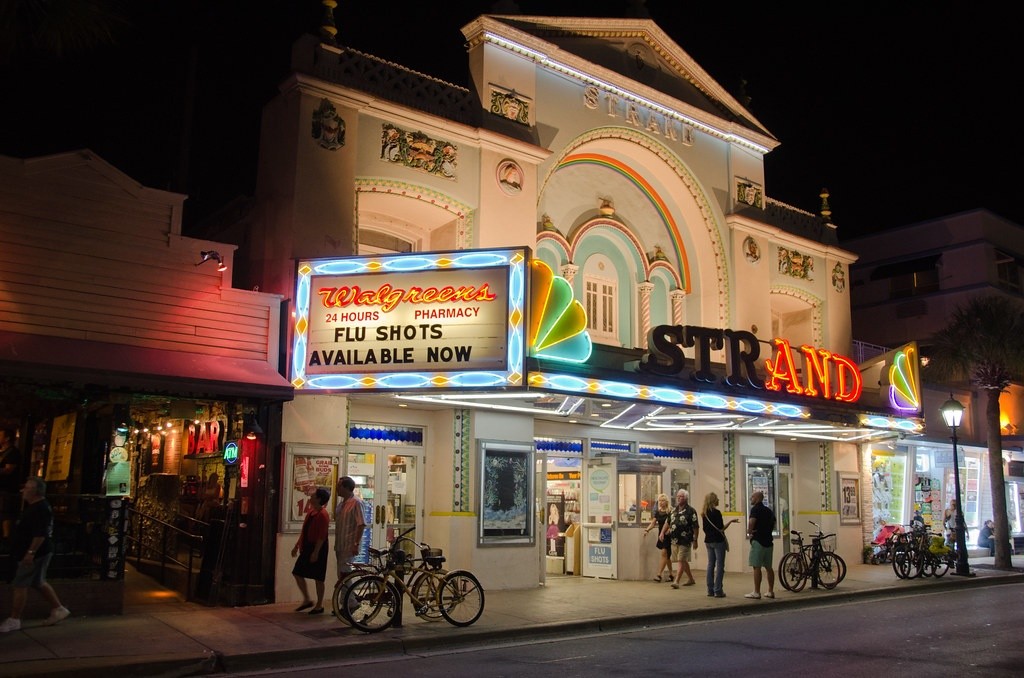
28, 551, 35, 554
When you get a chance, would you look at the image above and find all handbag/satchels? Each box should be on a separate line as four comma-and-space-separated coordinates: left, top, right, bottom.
725, 535, 730, 553
949, 532, 958, 542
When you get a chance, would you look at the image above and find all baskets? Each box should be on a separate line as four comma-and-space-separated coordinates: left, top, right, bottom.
819, 533, 836, 552
420, 547, 442, 561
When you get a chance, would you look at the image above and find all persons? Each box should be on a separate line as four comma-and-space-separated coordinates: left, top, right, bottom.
659, 489, 699, 588
744, 492, 777, 599
978, 520, 995, 557
701, 493, 740, 597
290, 488, 329, 616
943, 499, 969, 569
0, 427, 22, 544
197, 473, 220, 520
644, 494, 674, 582
331, 476, 365, 616
0, 477, 70, 633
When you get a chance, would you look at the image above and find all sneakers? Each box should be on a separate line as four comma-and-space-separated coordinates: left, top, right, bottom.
0, 618, 21, 632
40, 604, 71, 626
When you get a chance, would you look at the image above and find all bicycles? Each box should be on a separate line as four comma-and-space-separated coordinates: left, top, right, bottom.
332, 526, 485, 633
870, 524, 952, 579
778, 521, 846, 593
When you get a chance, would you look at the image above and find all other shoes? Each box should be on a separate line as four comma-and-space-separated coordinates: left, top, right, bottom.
743, 592, 761, 599
332, 603, 362, 618
682, 578, 696, 586
715, 591, 726, 597
665, 575, 674, 582
654, 574, 662, 582
706, 591, 714, 596
294, 600, 315, 612
671, 583, 679, 588
308, 606, 325, 615
763, 591, 774, 598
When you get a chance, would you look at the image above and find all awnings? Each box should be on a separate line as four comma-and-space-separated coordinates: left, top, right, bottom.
870, 253, 943, 282
993, 249, 1024, 267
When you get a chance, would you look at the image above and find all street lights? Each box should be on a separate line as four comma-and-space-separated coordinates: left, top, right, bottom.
939, 391, 975, 576
